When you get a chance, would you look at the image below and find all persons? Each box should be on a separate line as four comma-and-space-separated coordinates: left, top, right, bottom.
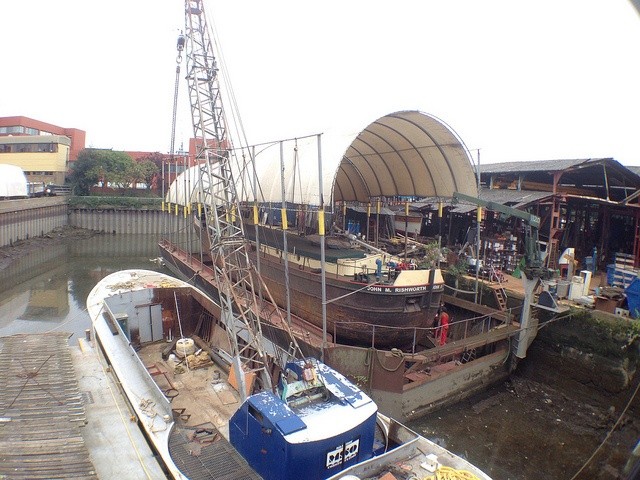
559, 247, 582, 275
440, 306, 449, 345
394, 260, 410, 277
595, 253, 607, 287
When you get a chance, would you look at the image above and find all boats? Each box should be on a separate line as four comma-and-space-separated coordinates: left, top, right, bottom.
157, 198, 519, 423
86, 269, 495, 480
